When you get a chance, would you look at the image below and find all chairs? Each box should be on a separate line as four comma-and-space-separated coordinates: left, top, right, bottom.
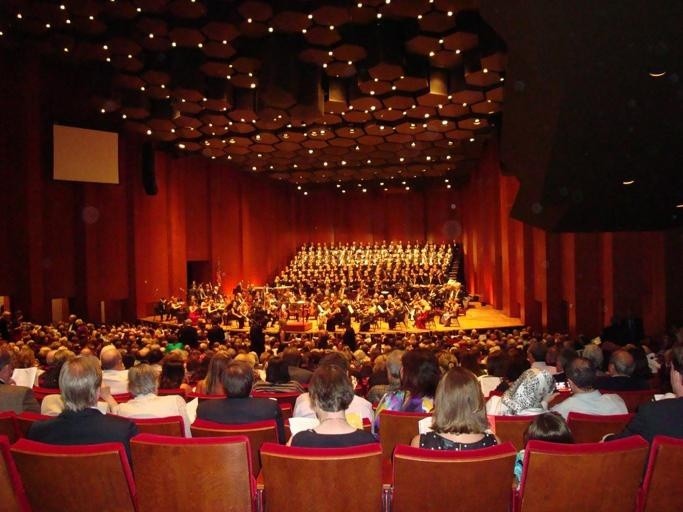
129, 432, 265, 508
283, 421, 292, 443
486, 412, 542, 453
13, 411, 58, 439
11, 437, 136, 508
261, 440, 386, 509
391, 442, 518, 508
566, 412, 635, 443
376, 409, 437, 462
106, 412, 187, 438
32, 379, 679, 411
2, 435, 31, 509
516, 435, 649, 508
2, 411, 18, 442
635, 436, 680, 508
189, 417, 281, 479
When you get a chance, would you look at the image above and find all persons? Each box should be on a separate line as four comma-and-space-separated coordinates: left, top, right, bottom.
0, 311, 682, 498
155, 239, 464, 331
